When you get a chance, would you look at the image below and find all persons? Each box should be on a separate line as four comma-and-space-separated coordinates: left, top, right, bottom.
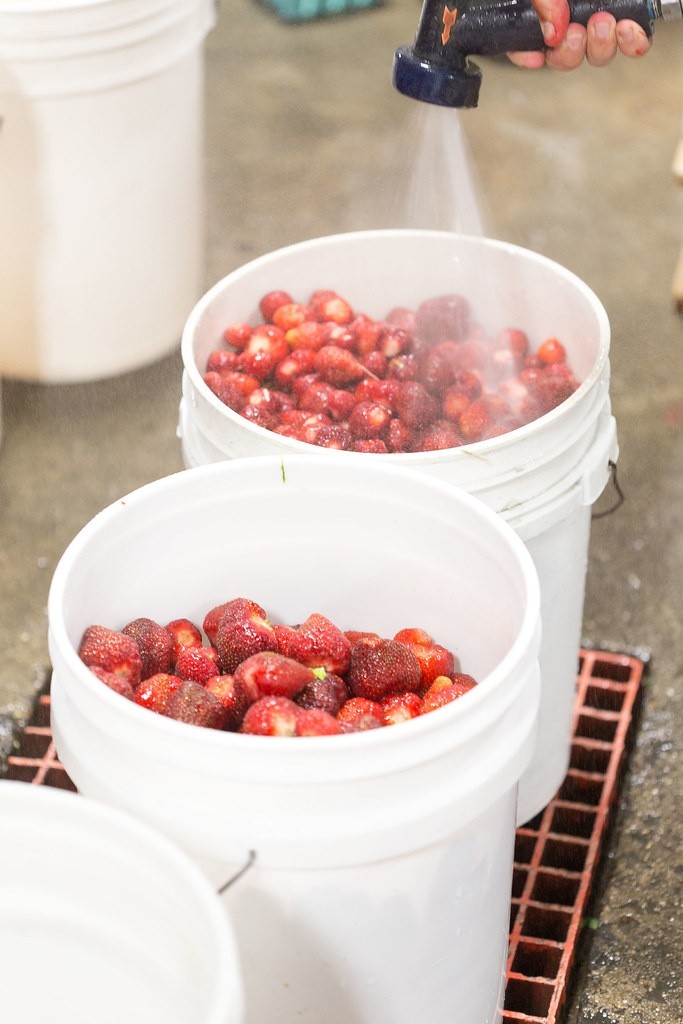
505, 0, 653, 71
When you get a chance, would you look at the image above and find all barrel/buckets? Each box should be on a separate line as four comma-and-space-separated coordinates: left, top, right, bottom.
183, 231, 624, 834
50, 455, 543, 1024
0, 781, 237, 1024
0, 0, 209, 386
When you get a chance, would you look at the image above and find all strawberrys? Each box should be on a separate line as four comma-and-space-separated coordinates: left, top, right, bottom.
199, 290, 583, 453
78, 597, 478, 738
